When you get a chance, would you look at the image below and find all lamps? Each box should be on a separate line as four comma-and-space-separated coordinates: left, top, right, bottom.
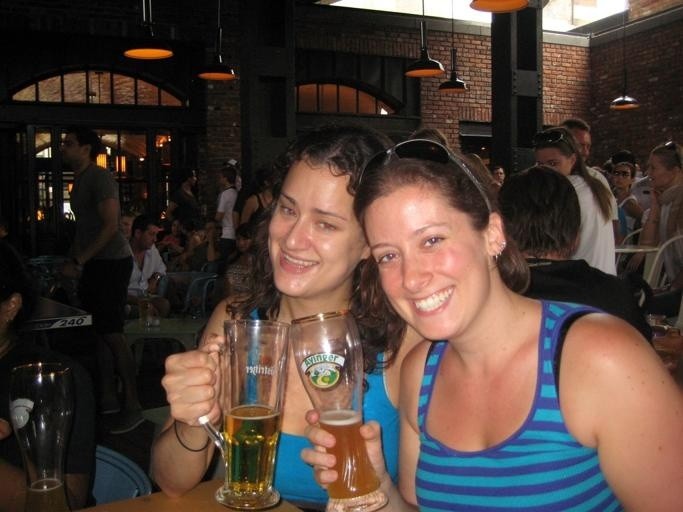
195, 0, 236, 80
122, 0, 174, 62
435, 0, 468, 94
404, 0, 446, 79
608, 13, 640, 110
469, 0, 529, 14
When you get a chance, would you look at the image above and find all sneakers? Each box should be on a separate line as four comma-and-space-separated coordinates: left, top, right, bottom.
109, 399, 148, 438
100, 389, 120, 415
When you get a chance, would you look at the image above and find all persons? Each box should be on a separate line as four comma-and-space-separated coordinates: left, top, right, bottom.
139, 122, 425, 512
292, 136, 682, 512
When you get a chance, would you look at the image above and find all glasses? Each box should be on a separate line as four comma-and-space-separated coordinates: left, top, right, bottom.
531, 130, 568, 146
614, 170, 630, 178
356, 137, 494, 217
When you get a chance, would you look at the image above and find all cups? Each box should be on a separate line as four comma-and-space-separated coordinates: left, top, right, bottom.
136, 287, 152, 330
287, 310, 389, 512
193, 318, 291, 508
7, 362, 77, 512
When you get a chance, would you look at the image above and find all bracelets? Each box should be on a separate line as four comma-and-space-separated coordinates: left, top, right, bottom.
168, 419, 212, 453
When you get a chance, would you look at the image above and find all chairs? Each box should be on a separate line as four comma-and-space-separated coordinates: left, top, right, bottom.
1, 251, 217, 512
640, 240, 682, 327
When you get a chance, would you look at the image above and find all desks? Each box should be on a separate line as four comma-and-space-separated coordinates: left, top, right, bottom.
81, 478, 302, 512
652, 334, 680, 377
0, 239, 682, 512
612, 244, 661, 254
